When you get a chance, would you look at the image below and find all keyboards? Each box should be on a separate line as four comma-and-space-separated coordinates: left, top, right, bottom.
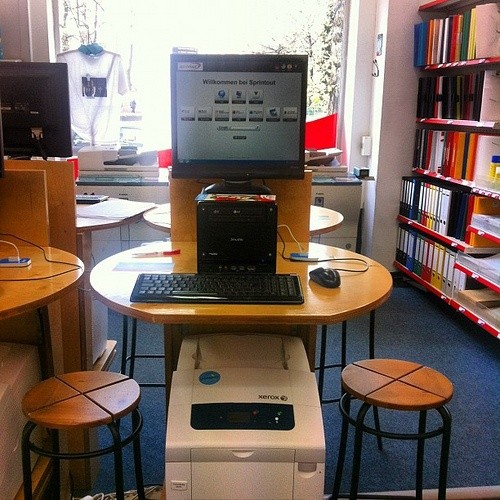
75, 193, 110, 206
129, 273, 306, 306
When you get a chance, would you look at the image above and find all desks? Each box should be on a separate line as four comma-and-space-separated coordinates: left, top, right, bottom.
90, 242, 393, 370
0, 243, 85, 500
145, 204, 343, 235
77, 198, 156, 360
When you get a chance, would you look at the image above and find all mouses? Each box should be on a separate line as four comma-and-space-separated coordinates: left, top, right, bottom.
308, 267, 342, 289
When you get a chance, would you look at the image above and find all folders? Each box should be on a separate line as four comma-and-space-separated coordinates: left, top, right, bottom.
396, 175, 466, 303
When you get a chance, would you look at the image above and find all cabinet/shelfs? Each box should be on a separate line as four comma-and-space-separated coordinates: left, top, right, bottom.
76, 174, 362, 267
394, 0, 500, 339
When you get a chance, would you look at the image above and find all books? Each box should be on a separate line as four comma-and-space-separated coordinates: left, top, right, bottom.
417, 69, 500, 122
447, 191, 500, 248
414, 128, 500, 183
414, 2, 500, 67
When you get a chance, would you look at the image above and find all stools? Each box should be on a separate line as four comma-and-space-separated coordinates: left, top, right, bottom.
331, 359, 455, 500
21, 371, 146, 500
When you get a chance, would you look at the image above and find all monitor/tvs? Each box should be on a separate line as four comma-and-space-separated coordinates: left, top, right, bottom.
172, 52, 307, 194
0, 61, 71, 160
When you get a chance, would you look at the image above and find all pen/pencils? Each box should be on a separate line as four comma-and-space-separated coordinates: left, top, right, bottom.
133, 248, 182, 256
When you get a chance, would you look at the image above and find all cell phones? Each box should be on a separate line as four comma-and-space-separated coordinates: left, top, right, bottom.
0, 256, 31, 268
290, 252, 319, 262
